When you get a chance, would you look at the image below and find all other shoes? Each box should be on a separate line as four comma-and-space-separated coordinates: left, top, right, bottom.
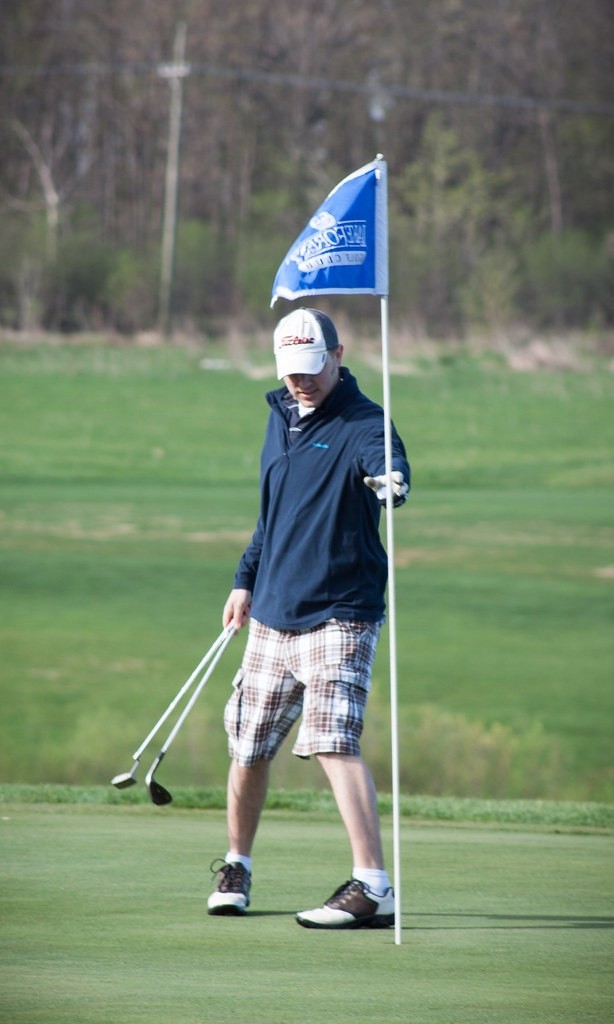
295, 880, 394, 929
206, 858, 252, 915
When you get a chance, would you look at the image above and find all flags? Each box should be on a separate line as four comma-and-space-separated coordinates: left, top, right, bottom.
269, 159, 391, 310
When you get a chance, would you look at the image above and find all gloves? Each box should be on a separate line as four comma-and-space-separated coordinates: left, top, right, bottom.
364, 470, 410, 499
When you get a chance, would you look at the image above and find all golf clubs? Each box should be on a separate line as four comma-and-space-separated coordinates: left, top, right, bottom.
143, 620, 239, 807
111, 616, 237, 790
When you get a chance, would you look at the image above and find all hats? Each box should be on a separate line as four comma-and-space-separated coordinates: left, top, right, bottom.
273, 307, 336, 380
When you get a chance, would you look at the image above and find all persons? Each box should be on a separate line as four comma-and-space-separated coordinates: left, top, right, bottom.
205, 306, 412, 929
